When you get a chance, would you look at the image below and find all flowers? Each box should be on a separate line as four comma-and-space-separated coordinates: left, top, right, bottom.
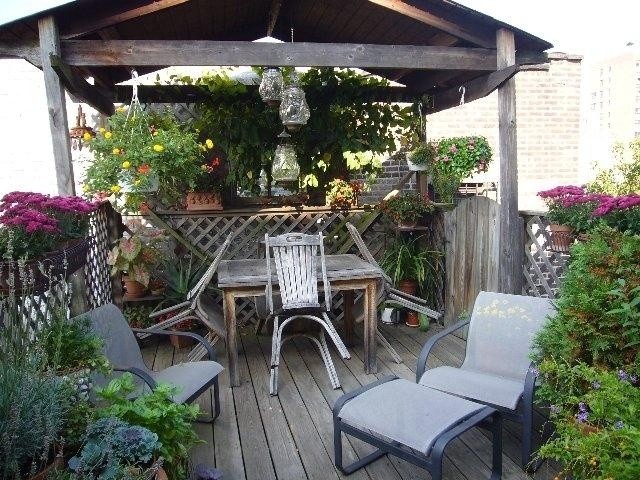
381, 133, 491, 230
536, 184, 640, 237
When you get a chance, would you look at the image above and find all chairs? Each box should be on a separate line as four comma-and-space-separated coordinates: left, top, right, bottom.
417, 290, 569, 473
64, 306, 221, 451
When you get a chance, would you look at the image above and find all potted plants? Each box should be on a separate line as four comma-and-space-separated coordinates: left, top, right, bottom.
0, 302, 208, 479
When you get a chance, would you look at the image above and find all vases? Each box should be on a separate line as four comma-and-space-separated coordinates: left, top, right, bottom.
549, 223, 604, 252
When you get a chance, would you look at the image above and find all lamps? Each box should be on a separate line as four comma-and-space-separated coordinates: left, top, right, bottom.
259, 67, 311, 187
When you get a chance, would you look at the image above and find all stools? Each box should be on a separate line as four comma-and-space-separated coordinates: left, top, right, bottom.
333, 376, 501, 480
